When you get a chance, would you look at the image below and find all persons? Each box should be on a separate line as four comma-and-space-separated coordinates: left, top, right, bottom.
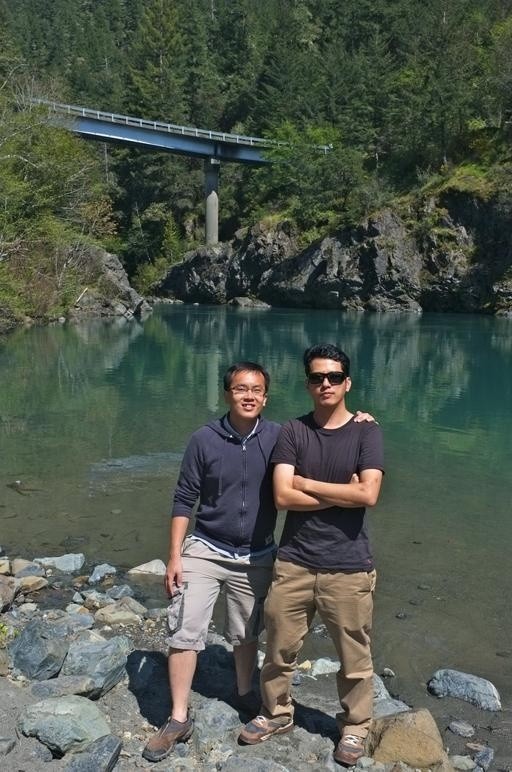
142, 359, 381, 763
238, 342, 385, 765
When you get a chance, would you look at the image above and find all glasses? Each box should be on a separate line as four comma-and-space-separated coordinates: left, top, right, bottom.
309, 371, 346, 384
228, 385, 267, 394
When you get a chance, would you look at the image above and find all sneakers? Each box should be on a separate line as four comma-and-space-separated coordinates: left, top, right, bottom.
240, 707, 294, 743
238, 691, 260, 722
334, 733, 367, 766
142, 707, 194, 762
